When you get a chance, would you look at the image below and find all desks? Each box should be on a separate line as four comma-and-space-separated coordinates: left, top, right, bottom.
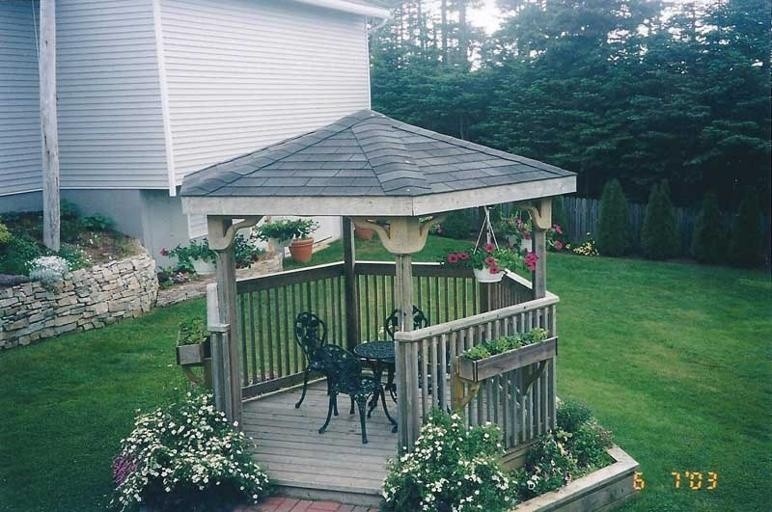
354, 341, 398, 425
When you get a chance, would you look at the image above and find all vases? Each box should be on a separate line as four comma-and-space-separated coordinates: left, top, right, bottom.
473, 266, 505, 283
354, 220, 376, 240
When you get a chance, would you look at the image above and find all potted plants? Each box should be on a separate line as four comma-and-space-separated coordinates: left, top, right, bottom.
458, 328, 558, 382
288, 219, 321, 263
177, 316, 206, 366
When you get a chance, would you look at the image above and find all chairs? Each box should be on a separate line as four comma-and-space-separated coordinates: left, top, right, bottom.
318, 344, 398, 444
385, 305, 429, 396
293, 312, 353, 416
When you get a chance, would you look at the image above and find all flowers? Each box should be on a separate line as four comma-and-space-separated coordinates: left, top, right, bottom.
445, 244, 539, 273
